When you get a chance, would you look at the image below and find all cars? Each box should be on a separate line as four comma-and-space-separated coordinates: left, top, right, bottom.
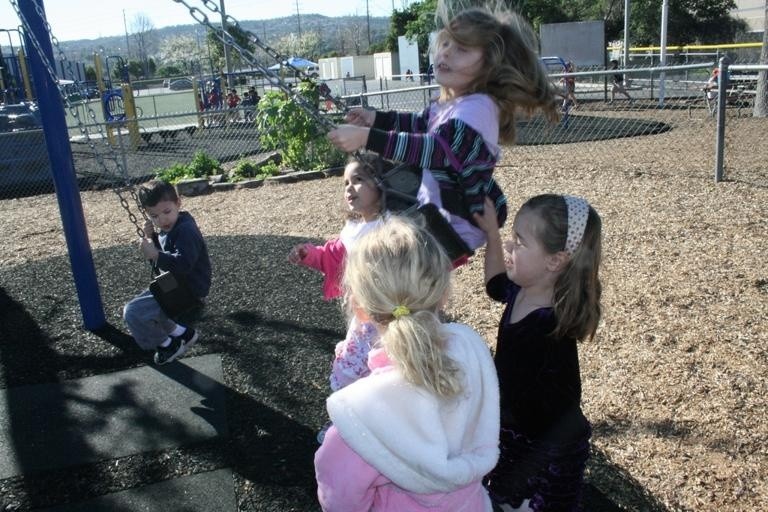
0, 101, 42, 133
163, 74, 246, 91
63, 87, 101, 104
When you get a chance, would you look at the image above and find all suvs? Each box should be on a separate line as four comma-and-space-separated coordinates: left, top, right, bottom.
304, 65, 320, 79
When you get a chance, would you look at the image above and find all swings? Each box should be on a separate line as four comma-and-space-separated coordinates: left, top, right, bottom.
176, 0, 475, 266
10, 0, 205, 322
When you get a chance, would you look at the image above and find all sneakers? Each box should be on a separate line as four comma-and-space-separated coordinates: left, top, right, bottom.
153, 326, 199, 366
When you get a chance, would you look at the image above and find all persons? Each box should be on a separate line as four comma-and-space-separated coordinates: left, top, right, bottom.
604, 59, 634, 105
287, 154, 392, 445
474, 195, 603, 511
326, 9, 564, 269
314, 217, 502, 511
197, 86, 261, 125
123, 180, 212, 365
